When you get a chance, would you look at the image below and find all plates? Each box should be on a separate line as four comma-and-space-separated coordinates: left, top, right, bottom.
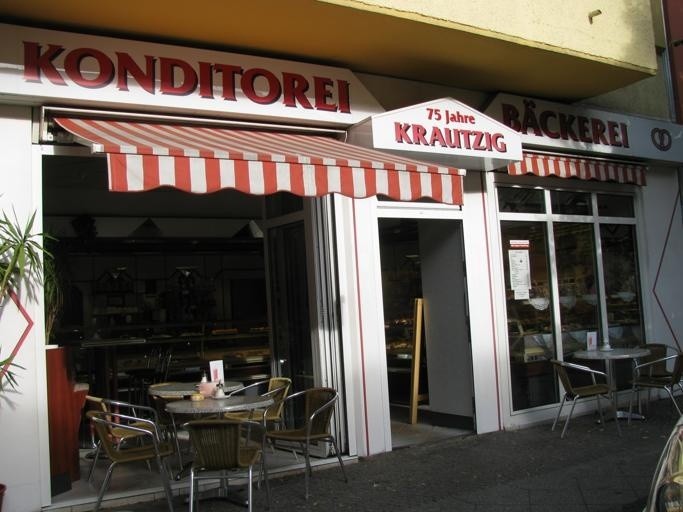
600, 349, 614, 352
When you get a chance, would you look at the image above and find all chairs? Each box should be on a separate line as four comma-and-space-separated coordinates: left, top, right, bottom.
549, 342, 682, 437
83, 374, 349, 512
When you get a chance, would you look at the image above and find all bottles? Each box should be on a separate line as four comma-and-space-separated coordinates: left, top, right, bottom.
214, 380, 223, 397
200, 369, 207, 383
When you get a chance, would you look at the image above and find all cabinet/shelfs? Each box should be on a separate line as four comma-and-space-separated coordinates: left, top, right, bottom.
507, 322, 646, 412
